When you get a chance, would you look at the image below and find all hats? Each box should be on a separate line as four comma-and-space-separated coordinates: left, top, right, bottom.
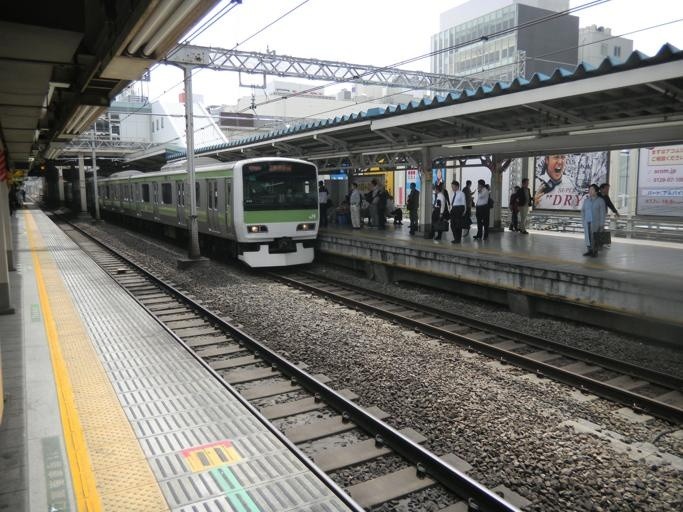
319, 181, 324, 186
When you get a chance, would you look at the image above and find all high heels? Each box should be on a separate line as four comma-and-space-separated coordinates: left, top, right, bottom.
426, 233, 442, 241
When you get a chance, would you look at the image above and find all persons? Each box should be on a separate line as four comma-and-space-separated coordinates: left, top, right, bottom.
318, 178, 419, 234
582, 184, 606, 257
534, 154, 579, 209
432, 169, 491, 244
516, 178, 534, 235
9, 188, 26, 216
597, 182, 620, 218
508, 186, 521, 232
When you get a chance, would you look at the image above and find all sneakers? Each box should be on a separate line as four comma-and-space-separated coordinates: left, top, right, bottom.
394, 221, 403, 225
451, 240, 461, 243
509, 226, 529, 235
582, 250, 598, 257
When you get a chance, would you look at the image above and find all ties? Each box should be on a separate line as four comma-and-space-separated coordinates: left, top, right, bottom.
451, 192, 456, 205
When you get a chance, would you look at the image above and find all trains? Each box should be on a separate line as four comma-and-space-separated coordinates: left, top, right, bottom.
63, 156, 321, 270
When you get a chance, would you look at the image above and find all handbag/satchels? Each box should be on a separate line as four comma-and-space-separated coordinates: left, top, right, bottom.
433, 221, 449, 231
489, 199, 493, 208
364, 192, 374, 204
593, 231, 611, 244
326, 199, 333, 209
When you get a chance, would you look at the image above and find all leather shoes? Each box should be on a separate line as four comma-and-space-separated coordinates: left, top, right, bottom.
473, 234, 482, 239
483, 236, 488, 240
352, 227, 360, 229
